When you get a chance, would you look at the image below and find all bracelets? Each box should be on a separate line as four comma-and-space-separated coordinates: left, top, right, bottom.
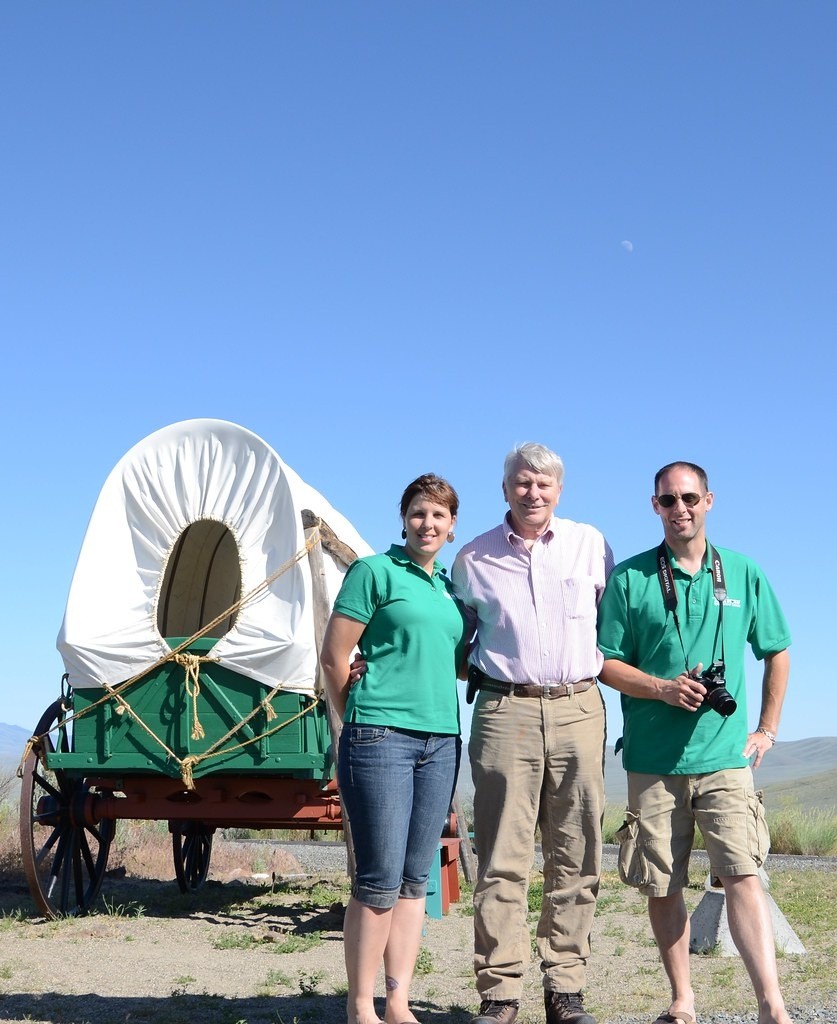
756, 729, 776, 745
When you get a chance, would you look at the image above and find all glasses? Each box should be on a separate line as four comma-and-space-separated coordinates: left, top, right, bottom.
656, 493, 708, 509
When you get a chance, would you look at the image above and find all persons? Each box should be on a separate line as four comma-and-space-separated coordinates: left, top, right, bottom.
351, 443, 616, 1024
598, 462, 795, 1024
320, 474, 467, 1024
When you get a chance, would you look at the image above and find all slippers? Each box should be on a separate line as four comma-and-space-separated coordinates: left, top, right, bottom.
651, 1011, 693, 1024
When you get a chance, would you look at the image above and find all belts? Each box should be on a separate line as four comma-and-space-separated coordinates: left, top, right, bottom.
477, 677, 596, 701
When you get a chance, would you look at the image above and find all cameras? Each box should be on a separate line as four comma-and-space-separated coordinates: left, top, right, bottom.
689, 662, 737, 718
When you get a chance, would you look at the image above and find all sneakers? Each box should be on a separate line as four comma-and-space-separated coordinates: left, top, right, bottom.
544, 988, 596, 1024
469, 998, 519, 1024
